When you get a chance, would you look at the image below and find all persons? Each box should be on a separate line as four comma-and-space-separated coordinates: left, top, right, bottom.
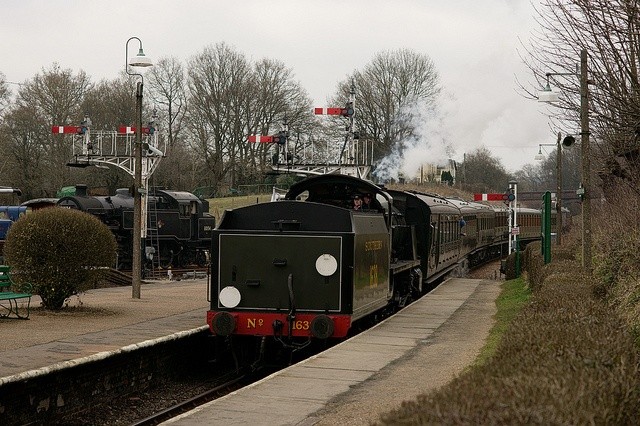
363, 193, 371, 208
353, 195, 362, 209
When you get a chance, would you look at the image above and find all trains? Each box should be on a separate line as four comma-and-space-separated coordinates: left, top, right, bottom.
205, 174, 572, 363
20, 184, 216, 272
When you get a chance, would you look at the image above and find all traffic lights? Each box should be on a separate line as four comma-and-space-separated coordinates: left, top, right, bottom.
341, 108, 353, 115
273, 134, 286, 143
502, 193, 515, 201
76, 127, 89, 133
143, 128, 156, 134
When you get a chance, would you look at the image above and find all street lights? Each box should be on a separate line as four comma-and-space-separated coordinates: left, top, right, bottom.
125, 36, 154, 300
535, 132, 562, 246
538, 48, 593, 268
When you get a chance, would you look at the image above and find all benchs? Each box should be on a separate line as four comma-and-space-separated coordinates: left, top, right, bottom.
0, 265, 33, 319
